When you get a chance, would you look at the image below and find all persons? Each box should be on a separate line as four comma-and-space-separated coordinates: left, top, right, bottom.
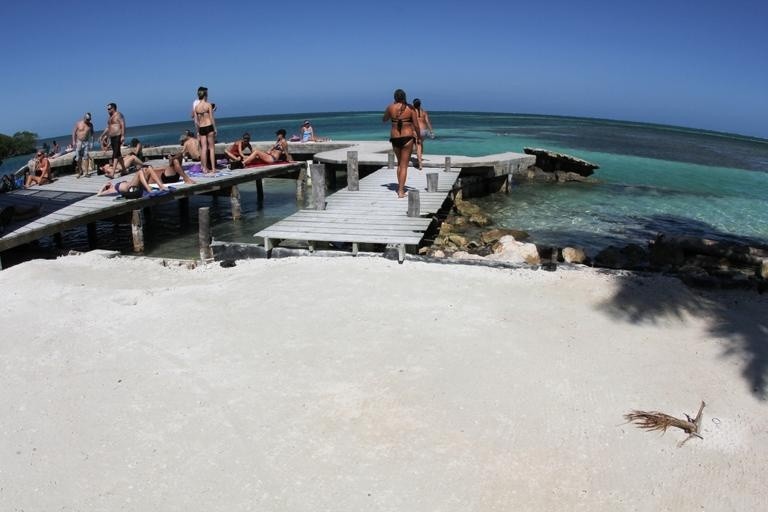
0, 86, 299, 200
300, 119, 331, 142
383, 88, 421, 198
411, 98, 436, 170
